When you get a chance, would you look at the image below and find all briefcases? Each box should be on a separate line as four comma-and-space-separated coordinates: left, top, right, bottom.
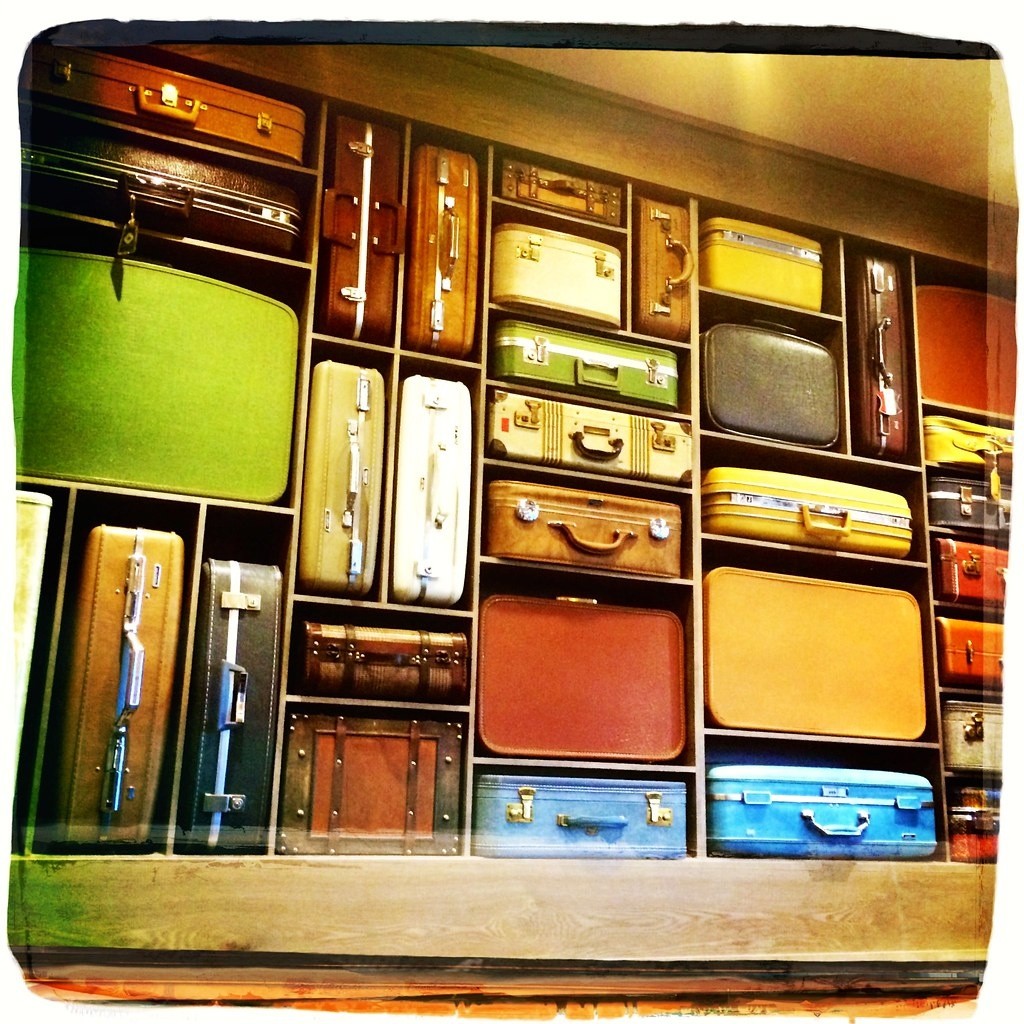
948, 785, 1000, 863
704, 762, 939, 861
930, 535, 1008, 610
298, 620, 469, 705
923, 413, 1014, 503
484, 219, 620, 332
17, 39, 306, 166
941, 698, 1003, 775
926, 476, 1011, 538
934, 615, 1004, 692
702, 565, 928, 743
843, 244, 912, 464
631, 193, 694, 344
476, 590, 688, 761
468, 772, 689, 861
483, 478, 685, 581
496, 157, 624, 228
489, 317, 680, 414
273, 708, 468, 856
389, 374, 473, 610
300, 358, 388, 600
12, 243, 301, 506
701, 465, 915, 560
16, 104, 308, 257
172, 554, 283, 852
319, 113, 407, 347
699, 216, 824, 312
698, 319, 841, 452
50, 523, 188, 850
918, 282, 1016, 414
485, 387, 693, 490
406, 141, 482, 360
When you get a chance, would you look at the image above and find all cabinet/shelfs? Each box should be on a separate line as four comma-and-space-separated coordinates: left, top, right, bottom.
15, 40, 1019, 863
